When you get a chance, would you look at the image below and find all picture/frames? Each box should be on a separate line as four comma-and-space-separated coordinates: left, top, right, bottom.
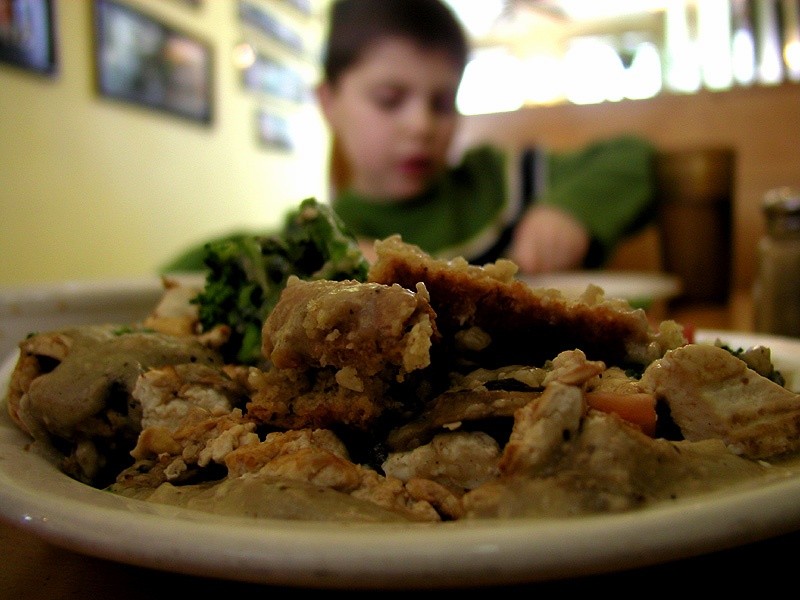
0, 0, 58, 80
91, 0, 215, 124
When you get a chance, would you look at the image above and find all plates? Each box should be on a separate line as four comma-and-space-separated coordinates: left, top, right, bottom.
2, 276, 680, 327
0, 328, 799, 589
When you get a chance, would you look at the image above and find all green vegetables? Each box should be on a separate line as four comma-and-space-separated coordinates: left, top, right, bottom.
189, 197, 370, 360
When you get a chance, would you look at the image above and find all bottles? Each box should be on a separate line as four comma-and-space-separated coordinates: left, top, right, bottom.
753, 187, 800, 329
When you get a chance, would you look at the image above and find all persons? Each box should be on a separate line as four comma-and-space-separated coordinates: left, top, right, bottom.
144, 0, 670, 273
